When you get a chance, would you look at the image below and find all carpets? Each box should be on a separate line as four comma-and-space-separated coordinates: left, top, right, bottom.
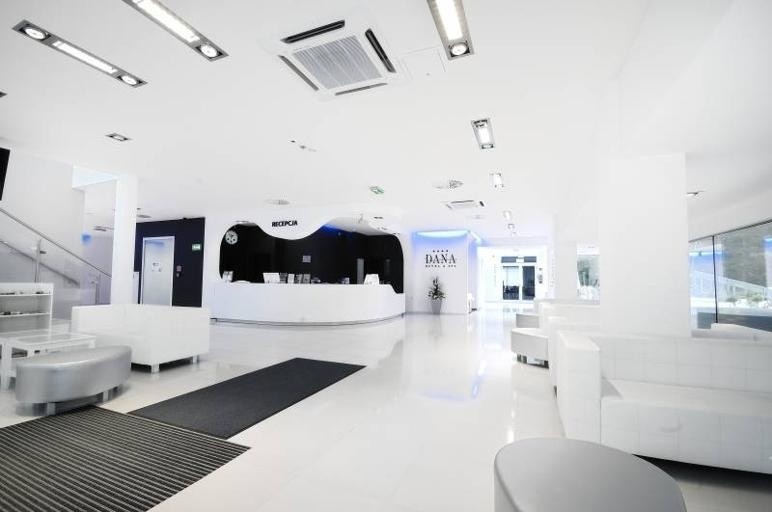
126, 356, 368, 440
0, 404, 252, 511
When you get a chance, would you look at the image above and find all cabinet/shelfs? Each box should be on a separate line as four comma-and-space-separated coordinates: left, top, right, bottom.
0, 282, 53, 329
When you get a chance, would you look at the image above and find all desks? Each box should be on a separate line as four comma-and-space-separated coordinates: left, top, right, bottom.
2, 334, 95, 391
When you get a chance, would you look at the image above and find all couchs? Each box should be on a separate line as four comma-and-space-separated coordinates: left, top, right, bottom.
545, 320, 772, 479
70, 305, 207, 372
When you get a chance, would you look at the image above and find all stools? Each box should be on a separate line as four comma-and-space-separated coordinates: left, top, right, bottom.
495, 306, 689, 512
14, 346, 132, 416
